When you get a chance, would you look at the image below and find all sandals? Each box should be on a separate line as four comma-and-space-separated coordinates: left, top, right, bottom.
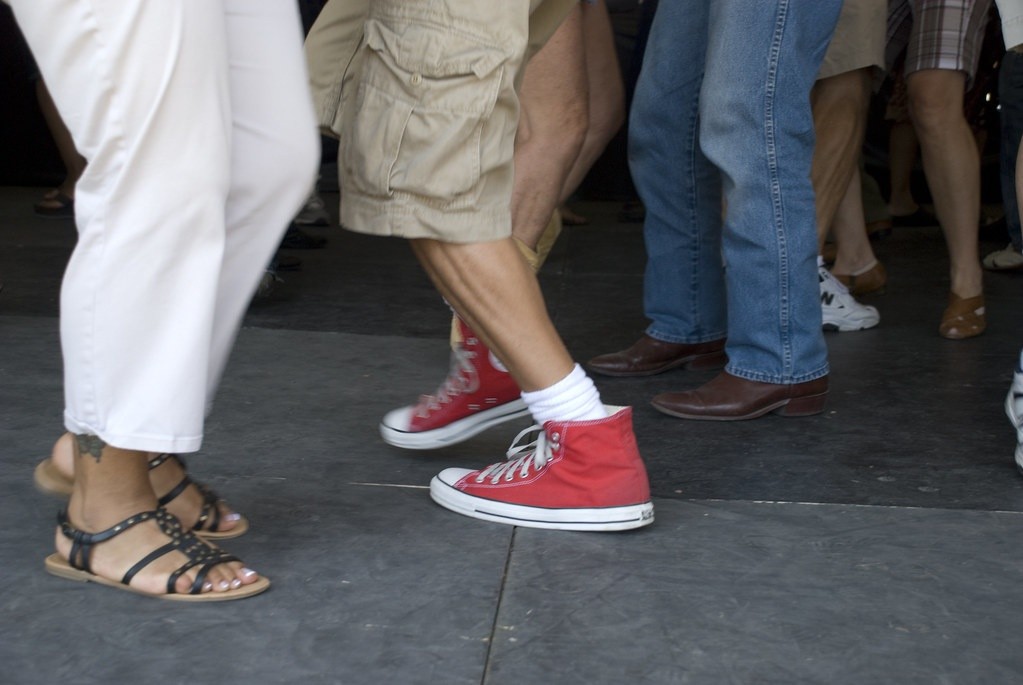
33, 184, 73, 216
43, 505, 270, 603
33, 454, 249, 540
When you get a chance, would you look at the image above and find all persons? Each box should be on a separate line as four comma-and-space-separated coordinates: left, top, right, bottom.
582, 0, 852, 422
2, 0, 319, 603
852, 2, 1023, 275
301, 0, 657, 539
721, 0, 882, 336
30, 63, 346, 308
447, 0, 638, 375
828, 1, 991, 343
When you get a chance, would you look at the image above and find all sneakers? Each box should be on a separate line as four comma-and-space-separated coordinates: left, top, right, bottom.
377, 311, 533, 450
816, 266, 881, 332
428, 404, 656, 532
1004, 364, 1023, 476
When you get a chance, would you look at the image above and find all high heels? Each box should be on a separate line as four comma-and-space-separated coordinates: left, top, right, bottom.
829, 260, 887, 301
938, 290, 988, 339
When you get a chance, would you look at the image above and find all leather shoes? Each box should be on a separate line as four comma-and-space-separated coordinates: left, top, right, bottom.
267, 251, 305, 271
866, 219, 893, 243
652, 370, 831, 421
280, 222, 327, 249
585, 331, 729, 376
821, 243, 838, 264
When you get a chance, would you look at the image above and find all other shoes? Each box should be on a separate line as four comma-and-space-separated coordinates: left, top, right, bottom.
981, 202, 1005, 225
982, 241, 1023, 269
890, 205, 944, 241
250, 271, 284, 308
